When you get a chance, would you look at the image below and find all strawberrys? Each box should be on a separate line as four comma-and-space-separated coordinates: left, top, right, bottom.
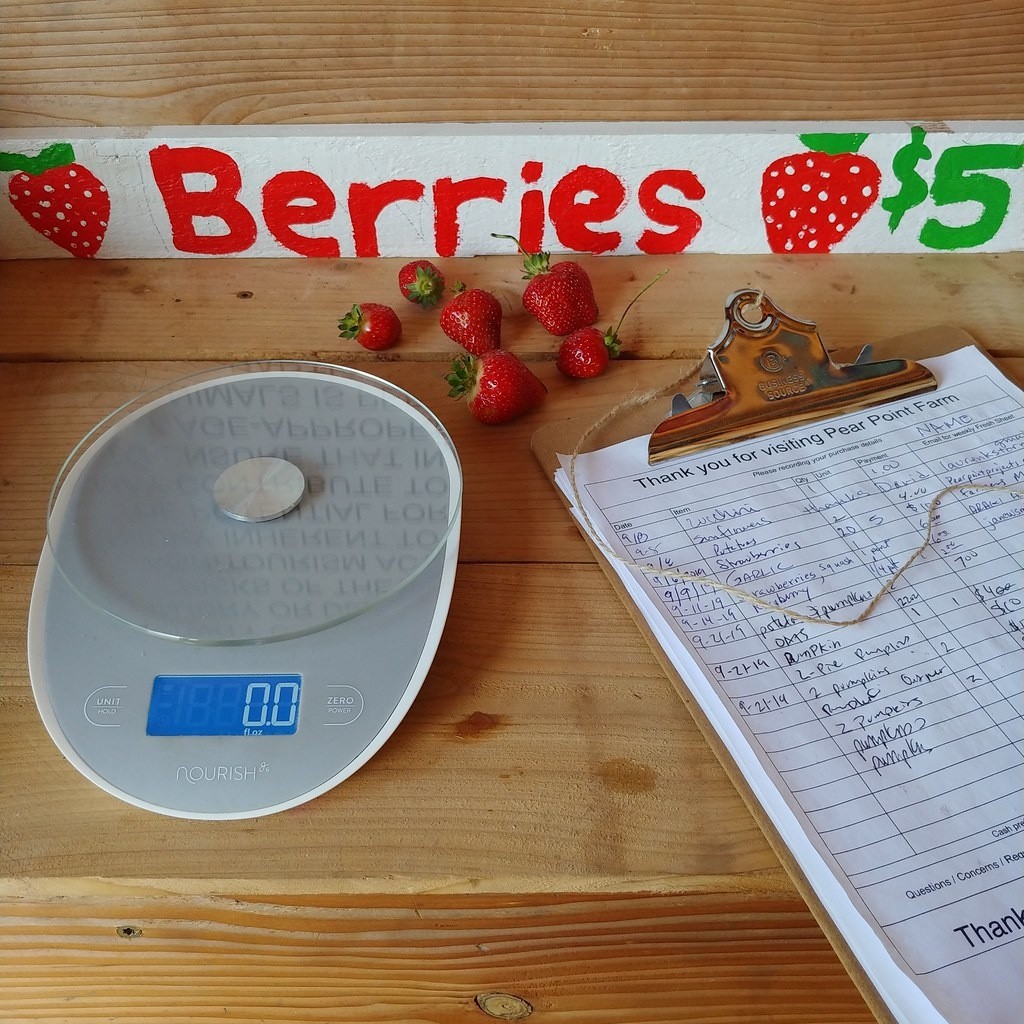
556, 325, 622, 380
440, 282, 501, 355
444, 348, 549, 427
521, 252, 600, 337
337, 302, 401, 350
398, 260, 446, 308
